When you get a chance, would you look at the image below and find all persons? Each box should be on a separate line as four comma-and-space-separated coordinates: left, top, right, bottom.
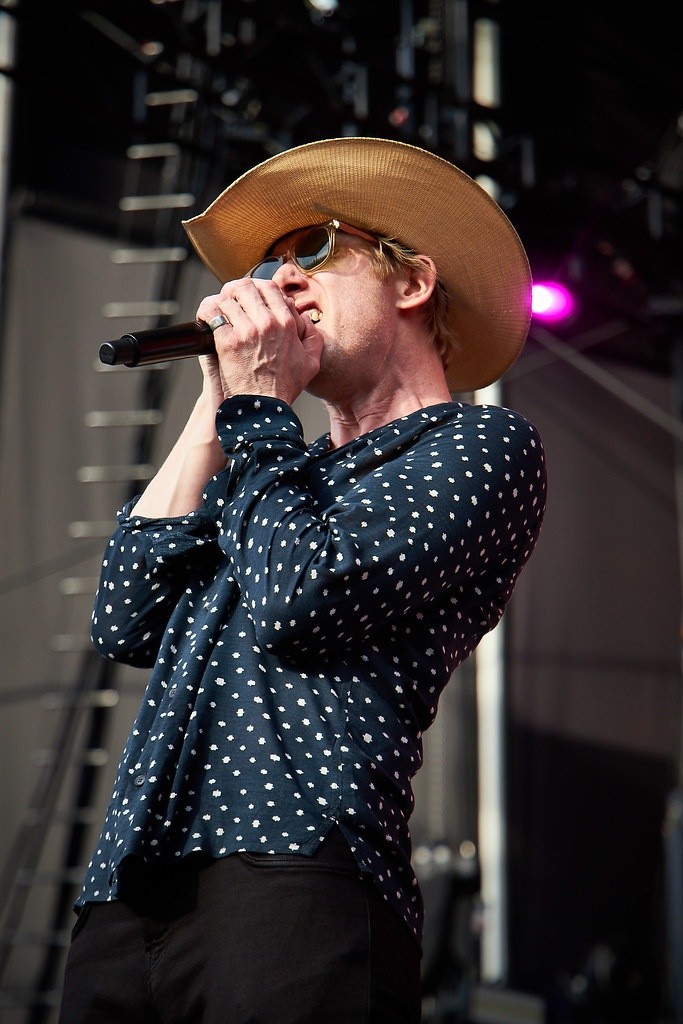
58, 134, 547, 1024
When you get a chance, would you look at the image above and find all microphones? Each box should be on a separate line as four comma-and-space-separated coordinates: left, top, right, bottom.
98, 321, 216, 370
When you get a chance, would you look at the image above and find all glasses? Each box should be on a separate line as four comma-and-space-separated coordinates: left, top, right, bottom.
240, 221, 420, 288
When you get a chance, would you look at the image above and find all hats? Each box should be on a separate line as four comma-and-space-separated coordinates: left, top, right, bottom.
176, 136, 538, 393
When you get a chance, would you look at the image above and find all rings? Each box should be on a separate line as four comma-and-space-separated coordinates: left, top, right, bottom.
208, 315, 229, 331
